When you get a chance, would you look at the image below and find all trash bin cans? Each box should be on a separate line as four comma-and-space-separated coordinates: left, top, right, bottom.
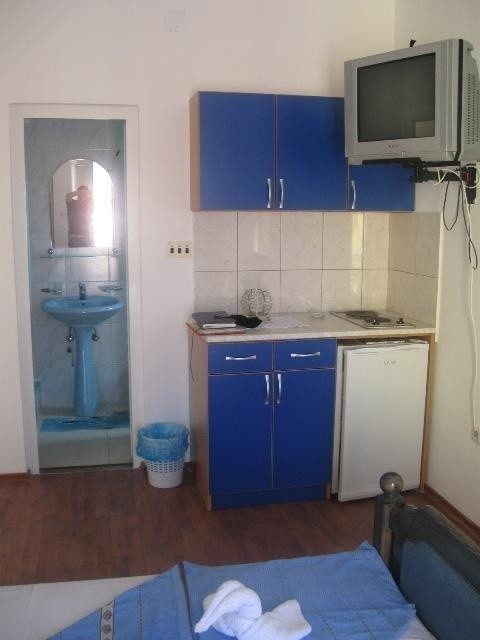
137, 421, 190, 489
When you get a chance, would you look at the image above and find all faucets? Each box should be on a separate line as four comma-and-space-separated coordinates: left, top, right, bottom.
78, 281, 88, 299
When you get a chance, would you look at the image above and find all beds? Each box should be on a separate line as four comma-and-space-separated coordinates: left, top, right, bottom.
0, 472, 479, 640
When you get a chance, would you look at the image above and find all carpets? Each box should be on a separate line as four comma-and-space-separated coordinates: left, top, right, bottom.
40, 411, 131, 433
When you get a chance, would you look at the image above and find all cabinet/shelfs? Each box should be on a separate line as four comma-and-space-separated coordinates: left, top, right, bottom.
348, 159, 416, 213
189, 91, 348, 213
185, 323, 338, 512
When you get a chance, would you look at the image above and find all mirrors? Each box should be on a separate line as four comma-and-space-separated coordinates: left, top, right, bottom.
51, 158, 115, 249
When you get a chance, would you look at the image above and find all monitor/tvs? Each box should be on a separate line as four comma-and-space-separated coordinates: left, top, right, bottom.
343, 37, 480, 168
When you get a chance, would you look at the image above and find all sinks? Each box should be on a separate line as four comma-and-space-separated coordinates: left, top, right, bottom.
42, 296, 123, 325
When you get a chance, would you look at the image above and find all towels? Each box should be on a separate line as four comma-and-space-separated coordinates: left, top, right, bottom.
193, 579, 312, 640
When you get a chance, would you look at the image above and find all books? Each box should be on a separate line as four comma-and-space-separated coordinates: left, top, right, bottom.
191, 309, 237, 329
